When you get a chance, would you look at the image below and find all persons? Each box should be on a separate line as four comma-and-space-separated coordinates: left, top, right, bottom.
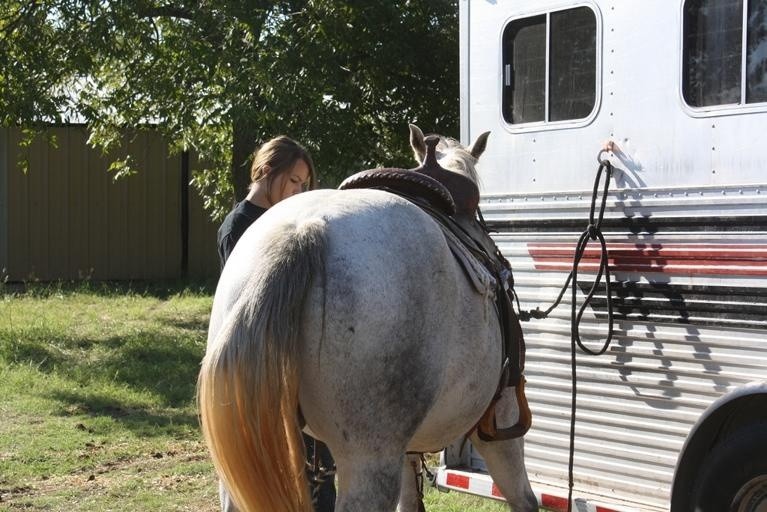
216, 134, 337, 512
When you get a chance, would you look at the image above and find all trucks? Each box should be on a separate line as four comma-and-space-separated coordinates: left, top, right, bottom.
434, 0, 767, 511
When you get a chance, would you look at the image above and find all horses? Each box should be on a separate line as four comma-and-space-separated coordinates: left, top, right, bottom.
194, 120, 541, 512
